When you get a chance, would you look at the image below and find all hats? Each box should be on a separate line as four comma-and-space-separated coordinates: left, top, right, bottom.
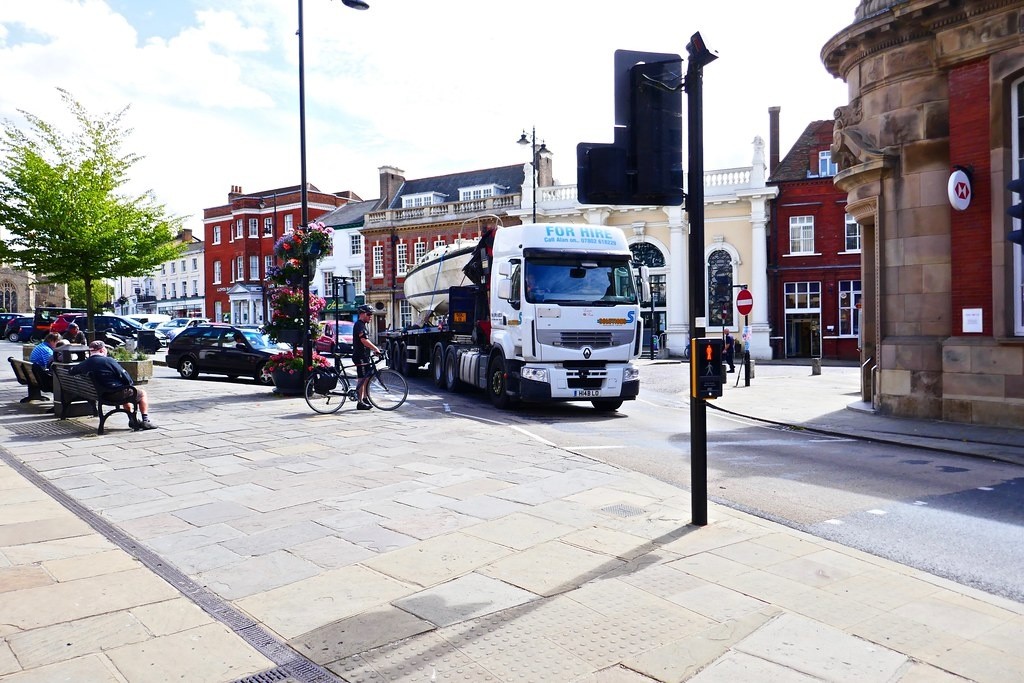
66, 323, 80, 332
89, 340, 106, 351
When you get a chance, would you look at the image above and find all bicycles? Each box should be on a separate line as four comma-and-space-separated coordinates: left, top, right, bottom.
304, 348, 408, 414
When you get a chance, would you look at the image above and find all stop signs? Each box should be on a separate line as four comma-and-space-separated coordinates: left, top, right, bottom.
737, 290, 753, 315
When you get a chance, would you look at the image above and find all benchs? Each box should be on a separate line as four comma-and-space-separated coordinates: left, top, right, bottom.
7, 357, 53, 404
51, 361, 140, 436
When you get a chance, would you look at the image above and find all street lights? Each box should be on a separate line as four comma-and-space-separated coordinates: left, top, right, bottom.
298, 0, 370, 395
517, 125, 550, 223
257, 193, 277, 293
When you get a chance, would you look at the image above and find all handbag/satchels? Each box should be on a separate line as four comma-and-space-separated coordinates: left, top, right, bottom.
312, 366, 342, 395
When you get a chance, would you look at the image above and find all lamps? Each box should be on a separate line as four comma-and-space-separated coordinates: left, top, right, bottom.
257, 199, 266, 209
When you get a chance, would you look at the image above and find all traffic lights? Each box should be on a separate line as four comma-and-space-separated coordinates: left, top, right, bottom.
691, 338, 723, 400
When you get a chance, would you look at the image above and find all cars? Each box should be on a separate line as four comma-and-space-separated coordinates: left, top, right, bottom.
65, 316, 141, 346
200, 321, 304, 357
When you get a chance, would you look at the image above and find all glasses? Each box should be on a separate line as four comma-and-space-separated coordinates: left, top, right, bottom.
363, 312, 373, 316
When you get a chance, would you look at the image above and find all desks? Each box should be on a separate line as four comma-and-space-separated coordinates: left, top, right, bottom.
54, 345, 94, 361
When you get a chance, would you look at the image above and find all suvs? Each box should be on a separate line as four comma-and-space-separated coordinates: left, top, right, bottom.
159, 317, 211, 339
166, 323, 290, 386
0, 314, 25, 338
5, 315, 34, 343
50, 314, 83, 334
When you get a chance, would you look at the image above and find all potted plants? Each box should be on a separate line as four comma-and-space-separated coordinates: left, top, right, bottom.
106, 347, 153, 384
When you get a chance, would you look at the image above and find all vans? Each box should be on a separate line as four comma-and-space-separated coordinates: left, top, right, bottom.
33, 307, 88, 341
315, 321, 355, 355
127, 314, 172, 324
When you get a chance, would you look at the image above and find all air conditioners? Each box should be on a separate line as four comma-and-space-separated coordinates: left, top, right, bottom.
371, 301, 388, 314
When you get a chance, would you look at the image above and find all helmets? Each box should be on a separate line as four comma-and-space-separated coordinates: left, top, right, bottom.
357, 305, 376, 313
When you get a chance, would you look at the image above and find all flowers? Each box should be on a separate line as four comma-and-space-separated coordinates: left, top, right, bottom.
256, 219, 337, 352
261, 349, 332, 382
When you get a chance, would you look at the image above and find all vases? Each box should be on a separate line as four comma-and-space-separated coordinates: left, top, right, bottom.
281, 303, 300, 317
285, 256, 318, 282
271, 368, 306, 396
276, 329, 300, 344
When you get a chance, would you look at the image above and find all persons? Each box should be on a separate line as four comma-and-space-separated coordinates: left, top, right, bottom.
68, 340, 158, 430
30, 331, 62, 376
722, 328, 735, 373
63, 323, 88, 347
351, 305, 381, 411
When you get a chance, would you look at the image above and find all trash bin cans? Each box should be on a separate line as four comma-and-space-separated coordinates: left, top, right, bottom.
137, 330, 155, 355
53, 343, 94, 417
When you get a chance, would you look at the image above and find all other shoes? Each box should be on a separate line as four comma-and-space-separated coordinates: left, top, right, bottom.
362, 397, 374, 407
128, 419, 144, 428
141, 421, 158, 429
726, 370, 734, 373
357, 401, 372, 410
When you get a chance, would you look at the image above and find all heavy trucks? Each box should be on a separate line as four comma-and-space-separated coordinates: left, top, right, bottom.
379, 214, 649, 411
